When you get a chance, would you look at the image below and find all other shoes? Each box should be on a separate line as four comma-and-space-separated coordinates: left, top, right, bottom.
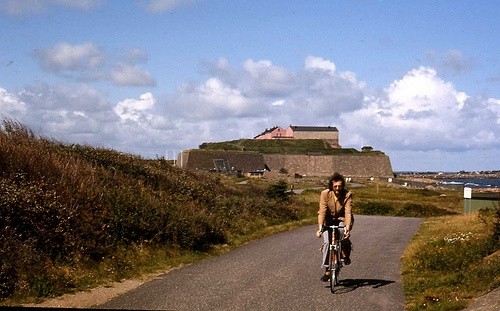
344, 257, 351, 265
320, 274, 330, 282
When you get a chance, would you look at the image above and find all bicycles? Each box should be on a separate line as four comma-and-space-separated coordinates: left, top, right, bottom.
321, 221, 348, 294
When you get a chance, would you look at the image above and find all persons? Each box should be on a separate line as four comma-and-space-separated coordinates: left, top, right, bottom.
316, 173, 355, 282
